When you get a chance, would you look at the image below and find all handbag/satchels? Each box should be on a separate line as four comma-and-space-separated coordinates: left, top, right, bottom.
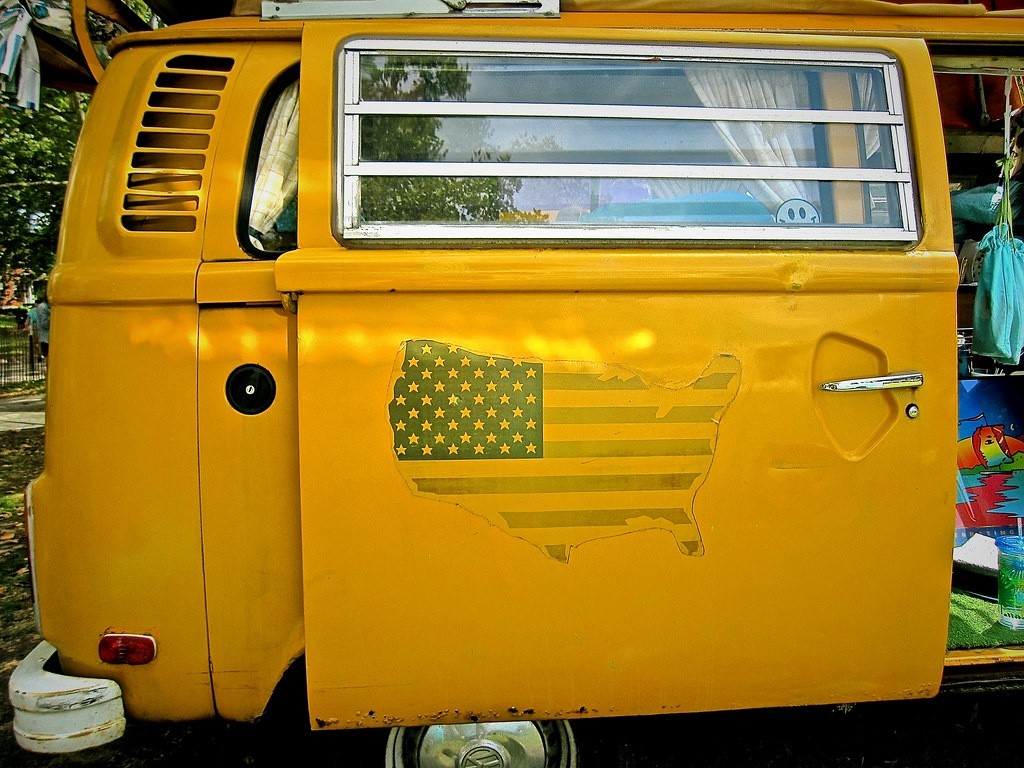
975, 224, 1023, 368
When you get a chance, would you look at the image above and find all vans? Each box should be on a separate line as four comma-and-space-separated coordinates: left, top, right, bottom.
8, 0, 1024, 768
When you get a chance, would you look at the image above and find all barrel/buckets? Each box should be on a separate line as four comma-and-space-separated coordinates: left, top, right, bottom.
995, 536, 1024, 630
